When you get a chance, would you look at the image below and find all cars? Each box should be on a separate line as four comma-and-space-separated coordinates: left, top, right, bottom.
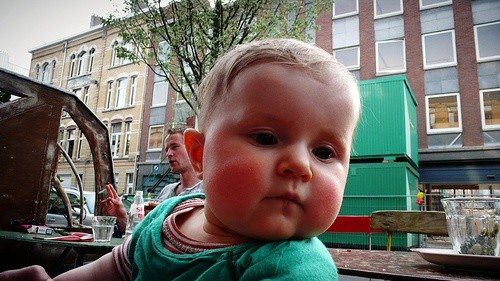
46, 187, 97, 226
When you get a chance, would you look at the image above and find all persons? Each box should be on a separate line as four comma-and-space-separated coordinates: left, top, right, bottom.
0, 38, 362, 281
416, 186, 426, 211
101, 127, 204, 233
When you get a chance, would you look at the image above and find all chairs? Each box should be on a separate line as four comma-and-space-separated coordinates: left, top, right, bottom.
326, 210, 449, 251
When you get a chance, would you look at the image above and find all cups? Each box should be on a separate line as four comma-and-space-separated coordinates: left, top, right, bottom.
440, 196, 500, 257
91, 216, 117, 242
125, 211, 145, 238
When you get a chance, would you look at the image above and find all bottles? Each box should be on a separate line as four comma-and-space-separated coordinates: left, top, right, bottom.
130, 191, 144, 220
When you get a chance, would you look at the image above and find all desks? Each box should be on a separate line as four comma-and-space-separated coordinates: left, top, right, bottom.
0, 230, 123, 281
326, 247, 500, 281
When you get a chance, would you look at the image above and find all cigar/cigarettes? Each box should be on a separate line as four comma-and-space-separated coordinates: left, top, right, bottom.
99, 188, 106, 194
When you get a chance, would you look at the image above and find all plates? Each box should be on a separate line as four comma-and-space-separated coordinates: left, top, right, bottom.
411, 248, 500, 272
